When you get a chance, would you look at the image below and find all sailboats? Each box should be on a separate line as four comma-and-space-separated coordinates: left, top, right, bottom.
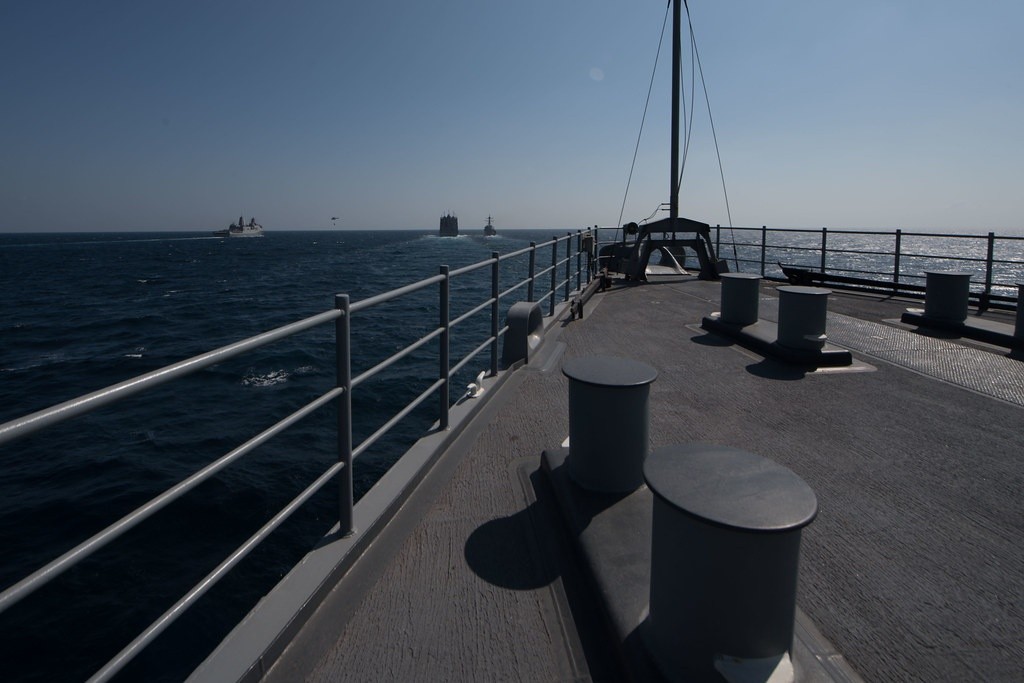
484, 213, 497, 236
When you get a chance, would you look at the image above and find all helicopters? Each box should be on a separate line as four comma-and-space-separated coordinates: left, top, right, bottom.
331, 217, 339, 220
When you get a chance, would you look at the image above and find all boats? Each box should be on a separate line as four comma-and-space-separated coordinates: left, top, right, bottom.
440, 209, 459, 237
229, 216, 265, 238
213, 229, 230, 237
